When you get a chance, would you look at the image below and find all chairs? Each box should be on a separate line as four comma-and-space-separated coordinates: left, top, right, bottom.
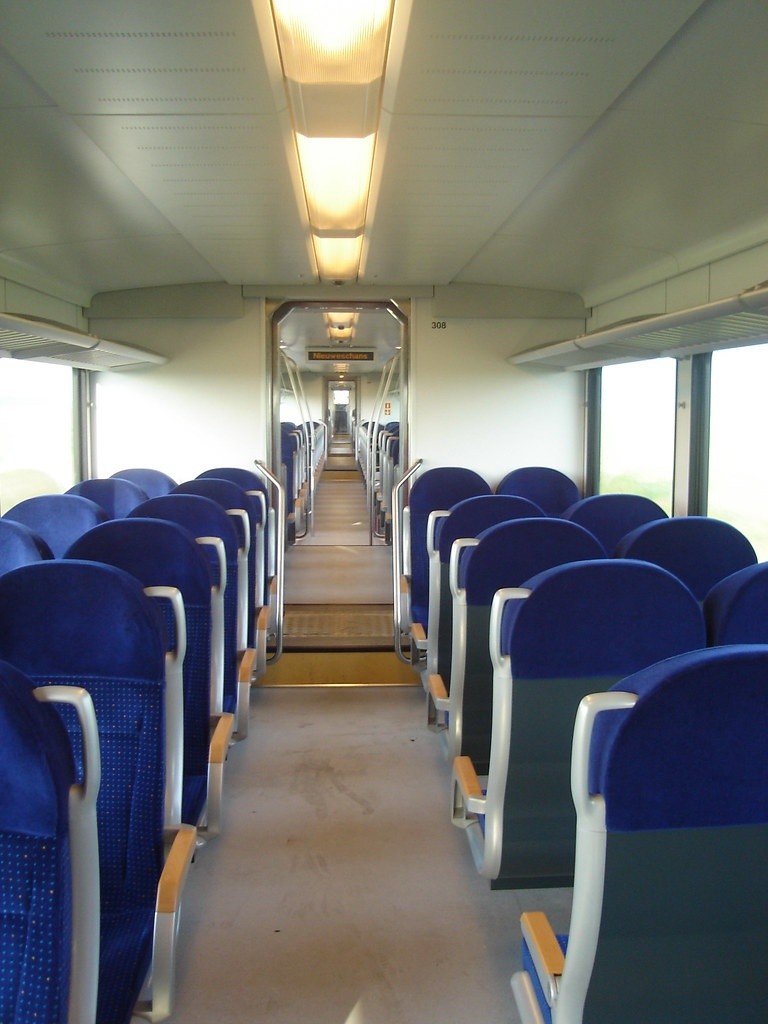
358, 420, 768, 1023
0, 422, 326, 1024
